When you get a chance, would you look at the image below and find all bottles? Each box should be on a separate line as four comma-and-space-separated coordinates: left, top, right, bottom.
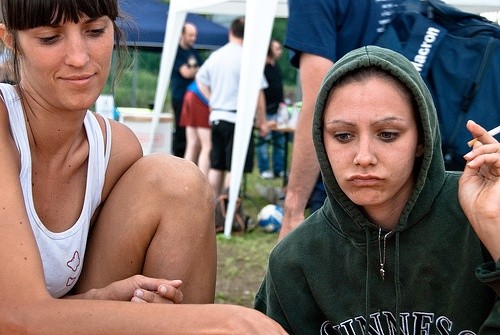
277, 102, 290, 129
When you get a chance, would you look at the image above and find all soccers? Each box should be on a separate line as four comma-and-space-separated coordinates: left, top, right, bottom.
258, 204, 285, 233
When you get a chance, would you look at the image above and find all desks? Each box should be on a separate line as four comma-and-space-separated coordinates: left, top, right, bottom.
253, 119, 295, 202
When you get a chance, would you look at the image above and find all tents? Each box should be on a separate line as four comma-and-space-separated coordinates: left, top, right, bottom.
110, 0, 231, 108
147, 0, 500, 239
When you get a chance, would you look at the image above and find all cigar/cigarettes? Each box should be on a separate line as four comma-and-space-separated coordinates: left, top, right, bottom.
468, 122, 500, 148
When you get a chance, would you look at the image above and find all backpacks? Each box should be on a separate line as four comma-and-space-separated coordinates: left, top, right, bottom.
214, 187, 249, 233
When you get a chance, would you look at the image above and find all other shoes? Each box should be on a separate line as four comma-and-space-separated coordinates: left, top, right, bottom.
255, 183, 286, 207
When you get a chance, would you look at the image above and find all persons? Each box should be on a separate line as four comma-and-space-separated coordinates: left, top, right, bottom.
254, 45, 500, 335
278, 0, 446, 238
168, 20, 287, 233
0, 0, 289, 335
